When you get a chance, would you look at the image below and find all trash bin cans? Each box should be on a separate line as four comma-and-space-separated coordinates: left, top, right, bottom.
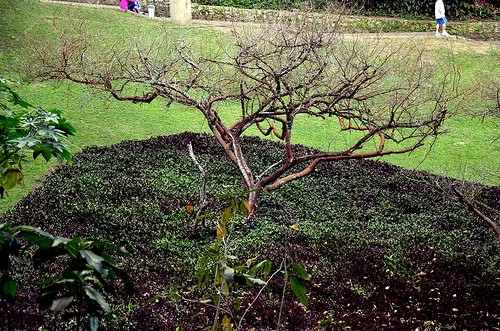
147, 4, 157, 20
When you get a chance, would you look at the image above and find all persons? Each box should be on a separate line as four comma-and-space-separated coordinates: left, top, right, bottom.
120, 0, 145, 16
434, 0, 450, 37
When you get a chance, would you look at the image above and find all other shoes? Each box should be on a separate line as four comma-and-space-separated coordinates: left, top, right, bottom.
442, 31, 450, 35
436, 32, 441, 35
